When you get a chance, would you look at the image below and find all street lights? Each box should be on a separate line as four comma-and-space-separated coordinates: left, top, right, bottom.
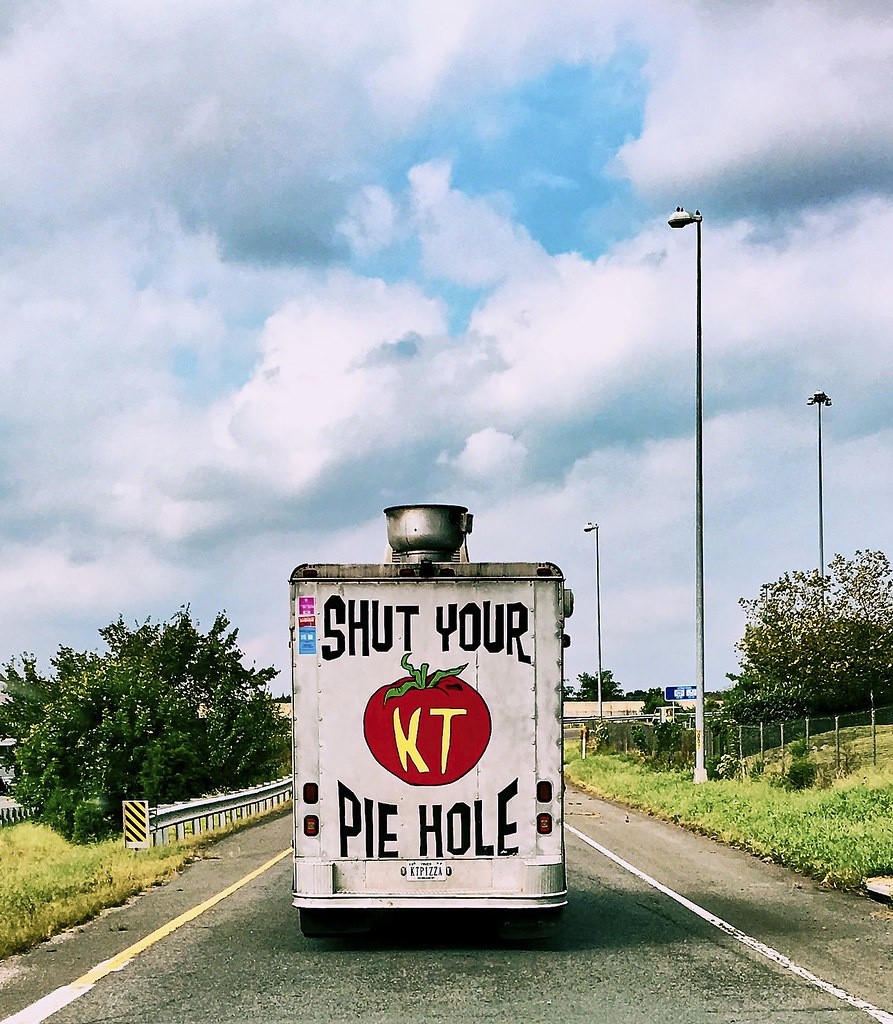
583, 522, 602, 723
668, 205, 707, 785
806, 390, 833, 609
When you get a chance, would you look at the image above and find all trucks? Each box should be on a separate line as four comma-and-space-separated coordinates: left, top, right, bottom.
288, 503, 574, 936
